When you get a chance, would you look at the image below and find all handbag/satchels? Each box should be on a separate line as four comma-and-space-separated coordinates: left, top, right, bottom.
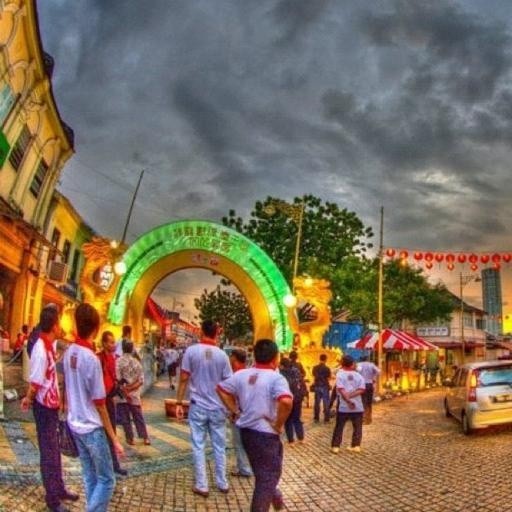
57, 356, 80, 458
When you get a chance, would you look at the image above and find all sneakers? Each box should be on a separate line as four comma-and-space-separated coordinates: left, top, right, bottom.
61, 488, 81, 502
217, 482, 231, 493
190, 483, 212, 497
346, 444, 366, 452
126, 437, 138, 445
329, 445, 339, 454
52, 502, 71, 512
144, 437, 150, 446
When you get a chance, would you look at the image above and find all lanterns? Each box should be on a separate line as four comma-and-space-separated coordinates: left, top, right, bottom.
385, 249, 511, 272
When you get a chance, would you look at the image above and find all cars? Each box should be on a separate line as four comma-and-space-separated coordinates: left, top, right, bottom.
496, 352, 511, 360
442, 360, 511, 435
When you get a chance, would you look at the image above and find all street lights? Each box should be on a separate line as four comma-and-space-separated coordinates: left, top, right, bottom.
460, 272, 481, 366
264, 198, 304, 296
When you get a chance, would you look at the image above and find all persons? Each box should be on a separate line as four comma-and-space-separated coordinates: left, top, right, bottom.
12, 303, 381, 511
